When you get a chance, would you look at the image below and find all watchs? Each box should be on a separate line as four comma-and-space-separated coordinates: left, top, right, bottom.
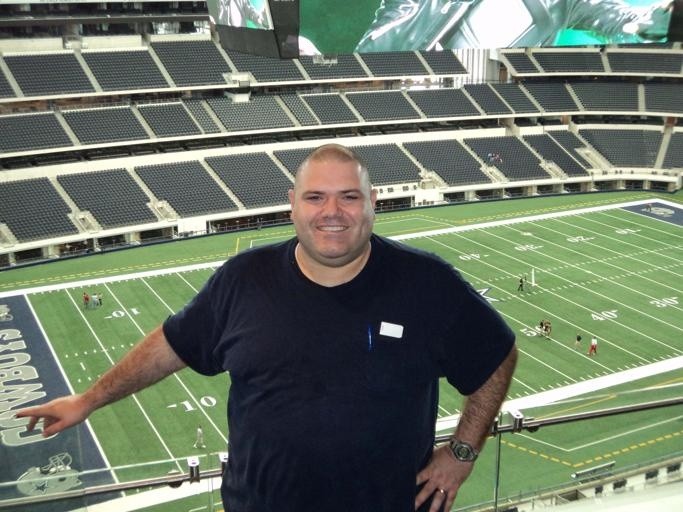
448, 432, 478, 467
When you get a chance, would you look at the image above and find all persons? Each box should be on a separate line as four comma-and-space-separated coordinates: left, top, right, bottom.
193, 424, 209, 449
82, 291, 104, 308
537, 318, 553, 337
12, 142, 519, 512
517, 278, 524, 292
574, 333, 584, 350
588, 335, 598, 357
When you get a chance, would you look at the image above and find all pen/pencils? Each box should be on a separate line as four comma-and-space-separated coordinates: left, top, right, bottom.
367, 323, 375, 353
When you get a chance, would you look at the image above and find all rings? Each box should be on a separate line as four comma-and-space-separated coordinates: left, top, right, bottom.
436, 487, 447, 495
